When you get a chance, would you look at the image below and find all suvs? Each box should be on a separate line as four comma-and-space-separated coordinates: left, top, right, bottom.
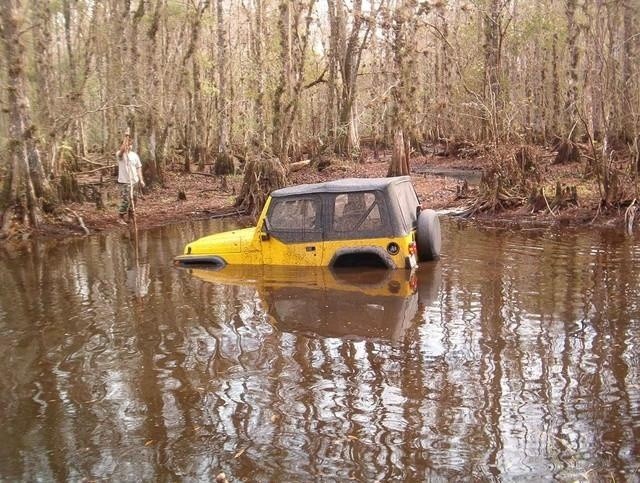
186, 263, 443, 343
173, 174, 443, 275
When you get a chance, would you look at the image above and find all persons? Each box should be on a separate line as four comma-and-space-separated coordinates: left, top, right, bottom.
116, 140, 145, 225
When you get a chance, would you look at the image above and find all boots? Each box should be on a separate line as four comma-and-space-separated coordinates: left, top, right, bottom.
117, 209, 133, 224
